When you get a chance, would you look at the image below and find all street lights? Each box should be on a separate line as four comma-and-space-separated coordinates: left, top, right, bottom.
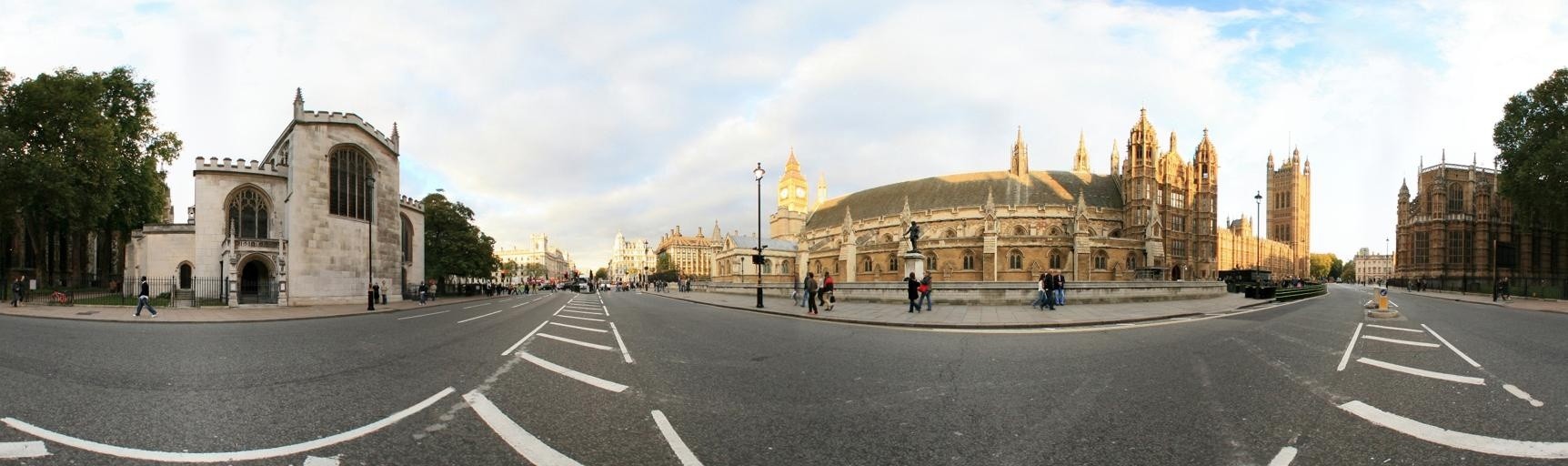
1385, 236, 1389, 287
1292, 239, 1305, 277
1254, 190, 1263, 299
751, 162, 768, 308
363, 173, 375, 311
643, 240, 649, 291
1362, 248, 1366, 286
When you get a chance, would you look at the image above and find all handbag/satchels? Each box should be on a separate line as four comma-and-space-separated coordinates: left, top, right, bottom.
917, 284, 929, 293
830, 296, 836, 303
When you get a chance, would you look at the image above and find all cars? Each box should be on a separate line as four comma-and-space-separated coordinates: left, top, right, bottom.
538, 275, 625, 292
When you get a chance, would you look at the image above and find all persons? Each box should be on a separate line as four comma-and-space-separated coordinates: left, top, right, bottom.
418, 281, 429, 305
1407, 277, 1428, 292
454, 280, 558, 297
904, 221, 920, 249
429, 280, 437, 300
903, 272, 922, 313
133, 276, 159, 317
373, 281, 380, 304
18, 275, 26, 302
380, 281, 389, 305
614, 278, 692, 293
10, 277, 21, 307
800, 271, 836, 316
920, 270, 933, 310
1498, 277, 1511, 301
1032, 268, 1065, 310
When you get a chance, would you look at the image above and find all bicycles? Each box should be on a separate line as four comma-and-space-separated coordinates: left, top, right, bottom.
50, 290, 73, 307
1498, 279, 1510, 301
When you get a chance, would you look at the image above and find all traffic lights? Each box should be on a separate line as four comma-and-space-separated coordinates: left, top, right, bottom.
501, 271, 505, 278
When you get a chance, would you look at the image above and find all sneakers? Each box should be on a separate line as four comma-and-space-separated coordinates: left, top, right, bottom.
800, 305, 818, 314
1032, 303, 1064, 311
819, 304, 834, 311
133, 313, 158, 317
908, 307, 932, 313
